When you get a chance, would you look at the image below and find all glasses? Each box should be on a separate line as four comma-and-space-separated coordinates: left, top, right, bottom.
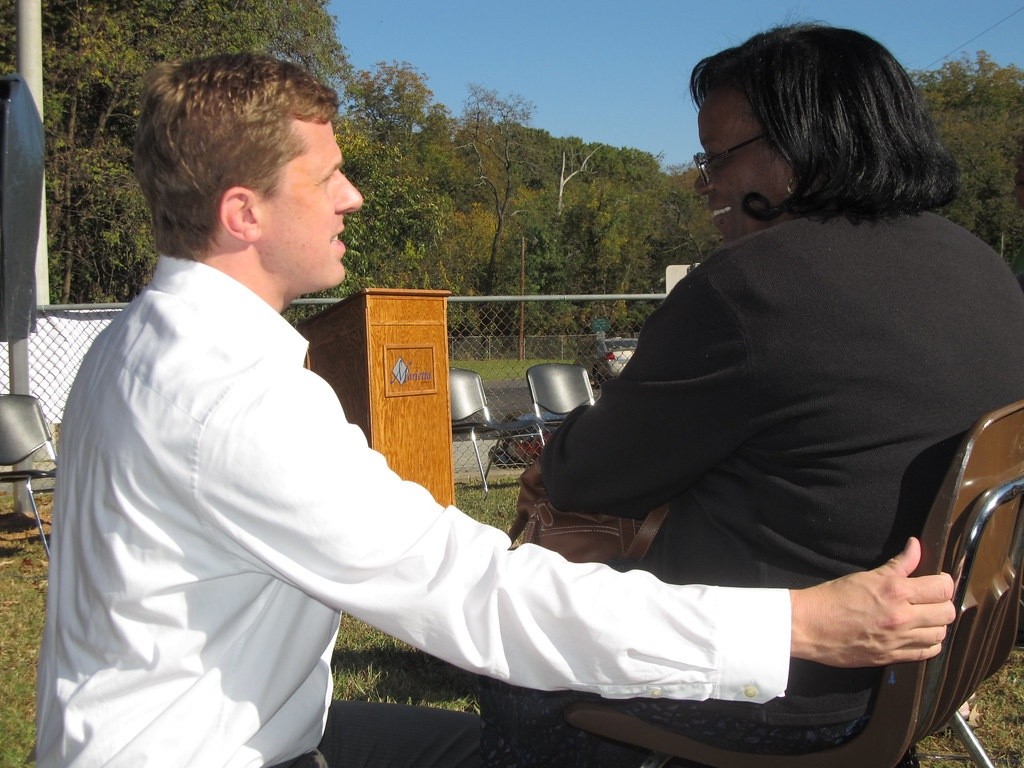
693, 132, 768, 185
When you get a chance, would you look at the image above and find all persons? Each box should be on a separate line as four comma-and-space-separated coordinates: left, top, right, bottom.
35, 52, 956, 768
538, 23, 1024, 768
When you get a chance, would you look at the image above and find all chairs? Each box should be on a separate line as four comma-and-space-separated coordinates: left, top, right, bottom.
526, 363, 596, 473
567, 399, 1024, 768
0, 394, 60, 560
448, 366, 547, 499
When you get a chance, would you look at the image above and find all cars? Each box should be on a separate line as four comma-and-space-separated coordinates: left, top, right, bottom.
572, 335, 640, 388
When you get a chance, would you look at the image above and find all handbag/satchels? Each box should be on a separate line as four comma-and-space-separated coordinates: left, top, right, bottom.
505, 457, 670, 572
